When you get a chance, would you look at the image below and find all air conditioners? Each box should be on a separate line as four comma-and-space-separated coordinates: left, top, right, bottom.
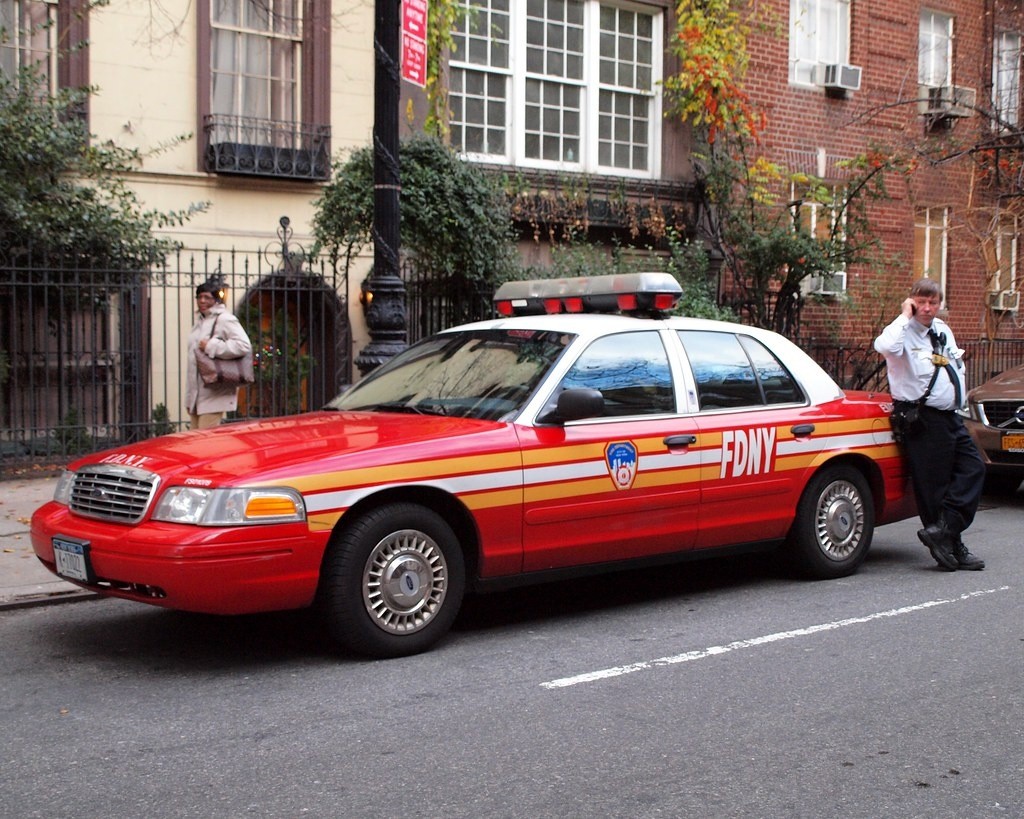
920, 84, 977, 117
808, 268, 847, 296
818, 62, 862, 92
989, 289, 1021, 312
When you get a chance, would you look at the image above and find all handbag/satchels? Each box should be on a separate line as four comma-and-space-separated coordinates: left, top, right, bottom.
194, 313, 254, 389
890, 397, 928, 435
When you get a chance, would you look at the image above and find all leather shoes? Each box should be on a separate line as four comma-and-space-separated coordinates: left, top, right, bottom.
954, 534, 985, 570
918, 515, 959, 572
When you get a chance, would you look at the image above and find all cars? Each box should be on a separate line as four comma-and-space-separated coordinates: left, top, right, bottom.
956, 364, 1023, 492
30, 271, 921, 657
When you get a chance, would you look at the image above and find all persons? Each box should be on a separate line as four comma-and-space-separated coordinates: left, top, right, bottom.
874, 278, 987, 571
184, 284, 252, 432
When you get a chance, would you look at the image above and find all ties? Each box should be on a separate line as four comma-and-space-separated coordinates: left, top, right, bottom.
927, 329, 962, 409
